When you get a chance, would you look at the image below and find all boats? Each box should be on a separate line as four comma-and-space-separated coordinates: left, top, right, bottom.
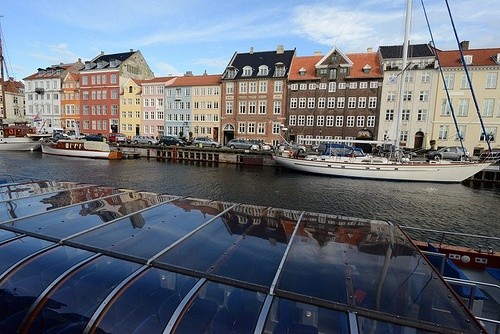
41, 138, 123, 160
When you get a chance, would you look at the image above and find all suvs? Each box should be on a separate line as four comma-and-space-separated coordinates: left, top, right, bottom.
424, 146, 469, 161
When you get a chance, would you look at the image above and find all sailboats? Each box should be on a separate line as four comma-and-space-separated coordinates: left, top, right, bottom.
0, 29, 44, 151
271, 0, 495, 183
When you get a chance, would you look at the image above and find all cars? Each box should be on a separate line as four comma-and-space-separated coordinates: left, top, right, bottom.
133, 136, 158, 145
193, 137, 223, 149
85, 134, 108, 142
109, 133, 127, 143
229, 140, 271, 151
160, 135, 187, 147
